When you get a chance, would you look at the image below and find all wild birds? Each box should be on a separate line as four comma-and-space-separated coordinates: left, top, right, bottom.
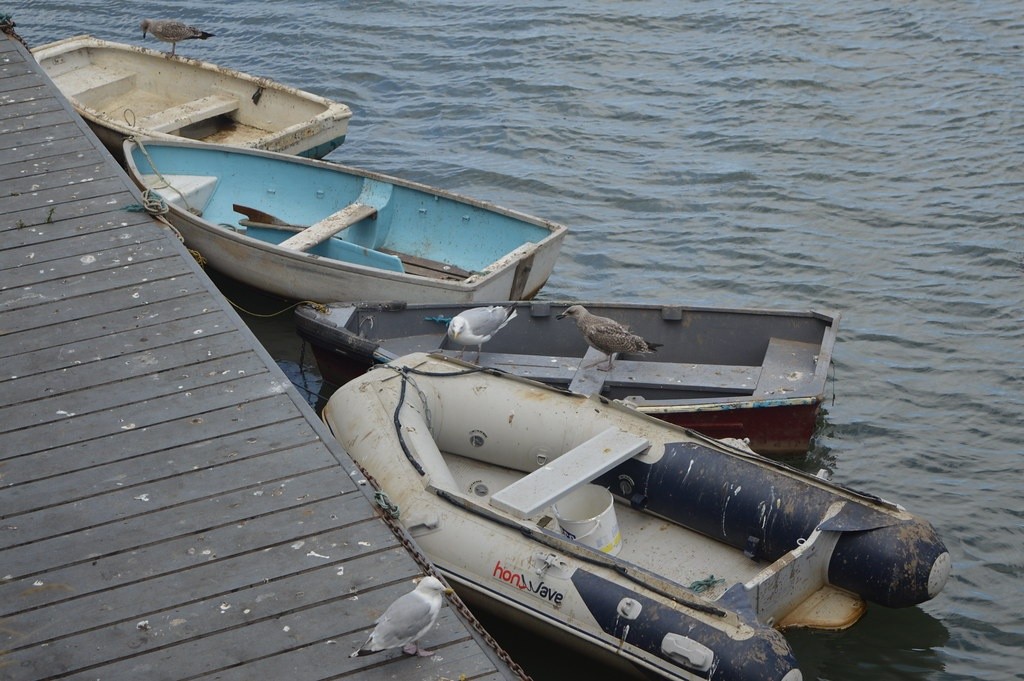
348, 576, 454, 658
448, 301, 520, 369
139, 18, 217, 60
556, 305, 664, 372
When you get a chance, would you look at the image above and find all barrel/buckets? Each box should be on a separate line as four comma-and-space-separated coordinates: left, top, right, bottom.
553, 485, 622, 557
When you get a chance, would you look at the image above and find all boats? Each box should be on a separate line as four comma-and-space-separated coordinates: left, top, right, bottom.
321, 351, 950, 681
123, 134, 569, 304
30, 35, 352, 160
292, 300, 842, 459
